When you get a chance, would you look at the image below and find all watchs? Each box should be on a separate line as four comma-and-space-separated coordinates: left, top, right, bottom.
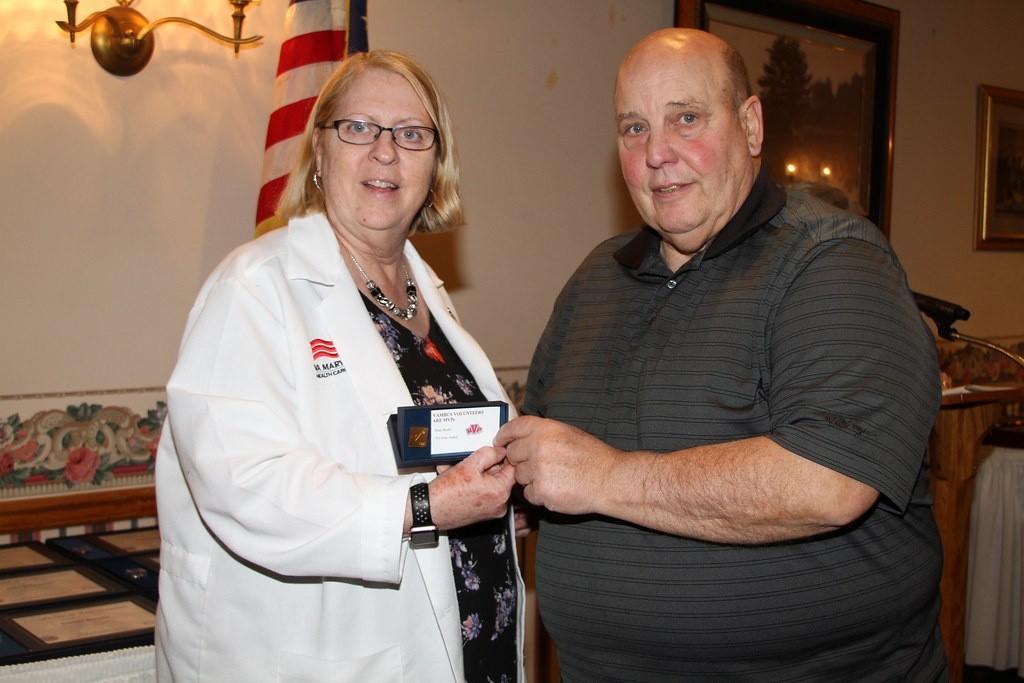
408, 482, 439, 549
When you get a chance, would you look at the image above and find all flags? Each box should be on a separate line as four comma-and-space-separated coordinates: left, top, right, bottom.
255, 0, 369, 240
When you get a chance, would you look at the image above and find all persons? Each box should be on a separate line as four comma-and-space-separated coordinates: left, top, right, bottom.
154, 50, 528, 683
492, 26, 945, 683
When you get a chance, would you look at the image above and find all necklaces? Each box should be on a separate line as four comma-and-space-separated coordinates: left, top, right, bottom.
345, 245, 420, 322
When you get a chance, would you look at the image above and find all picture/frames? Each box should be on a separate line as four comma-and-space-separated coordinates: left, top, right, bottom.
673, 0, 901, 251
971, 84, 1024, 251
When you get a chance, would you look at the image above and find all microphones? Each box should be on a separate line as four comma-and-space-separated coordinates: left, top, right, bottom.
909, 290, 970, 322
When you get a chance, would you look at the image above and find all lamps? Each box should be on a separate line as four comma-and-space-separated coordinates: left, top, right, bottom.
55, 0, 264, 76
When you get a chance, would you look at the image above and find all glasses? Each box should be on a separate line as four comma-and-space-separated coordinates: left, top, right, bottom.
322, 119, 439, 151
